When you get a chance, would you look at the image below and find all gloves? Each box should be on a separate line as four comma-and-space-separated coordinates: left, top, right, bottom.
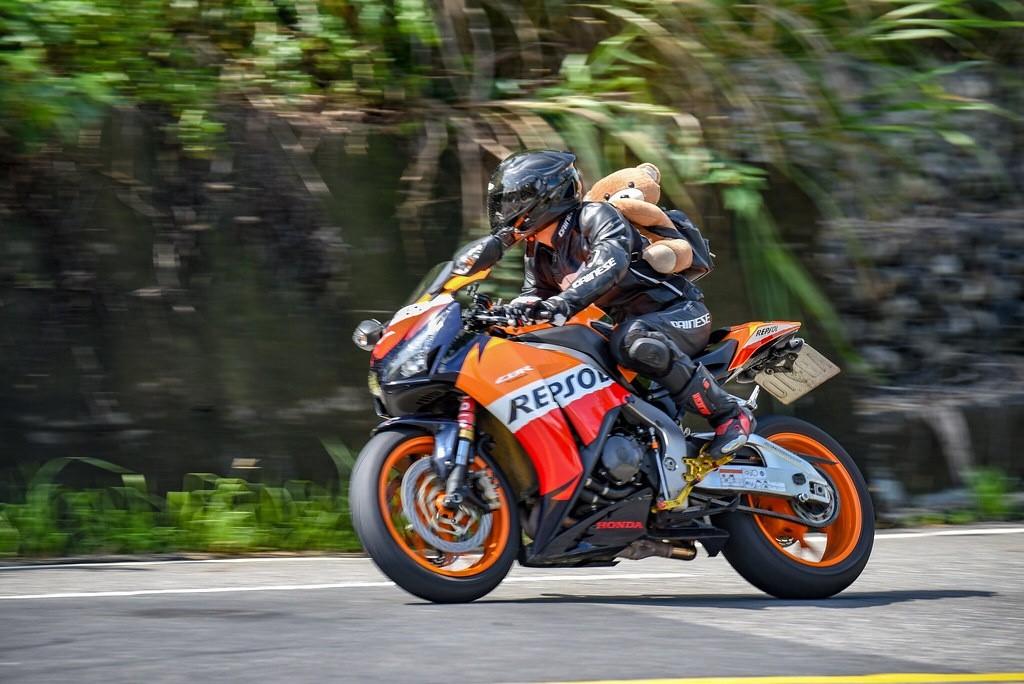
505, 295, 571, 331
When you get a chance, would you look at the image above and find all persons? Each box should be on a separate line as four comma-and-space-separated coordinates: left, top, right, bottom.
487, 149, 757, 459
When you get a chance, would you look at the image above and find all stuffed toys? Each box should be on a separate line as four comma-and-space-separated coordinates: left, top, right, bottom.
583, 162, 694, 273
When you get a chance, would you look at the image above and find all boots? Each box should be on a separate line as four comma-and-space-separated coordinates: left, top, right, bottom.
672, 362, 757, 459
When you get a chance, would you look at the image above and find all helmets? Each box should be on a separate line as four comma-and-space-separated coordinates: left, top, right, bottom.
487, 149, 581, 251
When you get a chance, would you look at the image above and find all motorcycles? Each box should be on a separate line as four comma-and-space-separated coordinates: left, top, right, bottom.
349, 235, 875, 605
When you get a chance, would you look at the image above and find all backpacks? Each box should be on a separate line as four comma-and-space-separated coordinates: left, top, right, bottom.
644, 205, 715, 283
